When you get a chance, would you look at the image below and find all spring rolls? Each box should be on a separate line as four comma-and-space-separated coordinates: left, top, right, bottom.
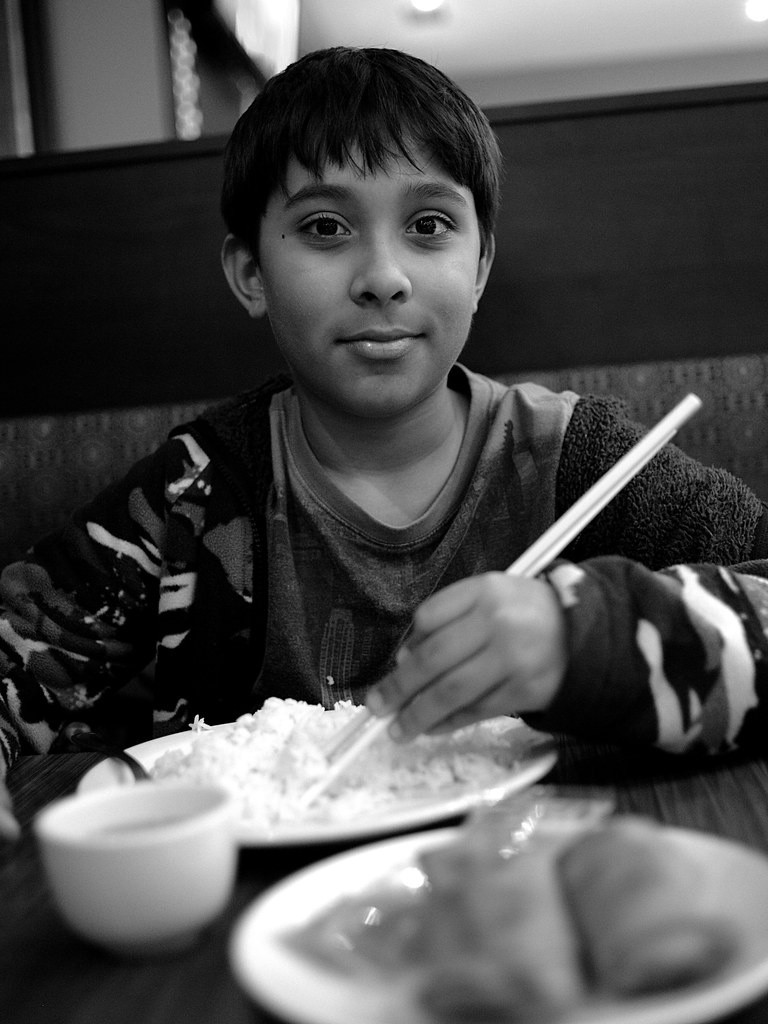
563, 810, 736, 993
410, 826, 589, 1024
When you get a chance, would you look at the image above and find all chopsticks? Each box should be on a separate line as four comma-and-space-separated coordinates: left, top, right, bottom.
296, 393, 701, 808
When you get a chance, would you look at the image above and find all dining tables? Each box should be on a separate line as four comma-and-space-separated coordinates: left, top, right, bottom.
0, 745, 766, 1023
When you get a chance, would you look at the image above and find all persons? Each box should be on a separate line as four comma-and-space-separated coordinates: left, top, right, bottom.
0, 46, 768, 842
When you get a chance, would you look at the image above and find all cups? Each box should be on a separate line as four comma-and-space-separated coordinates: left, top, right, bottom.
32, 784, 238, 944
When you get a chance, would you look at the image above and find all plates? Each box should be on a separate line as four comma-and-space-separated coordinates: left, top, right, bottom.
79, 710, 560, 846
231, 825, 768, 1024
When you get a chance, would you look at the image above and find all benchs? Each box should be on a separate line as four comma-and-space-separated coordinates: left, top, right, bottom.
0, 355, 767, 574
